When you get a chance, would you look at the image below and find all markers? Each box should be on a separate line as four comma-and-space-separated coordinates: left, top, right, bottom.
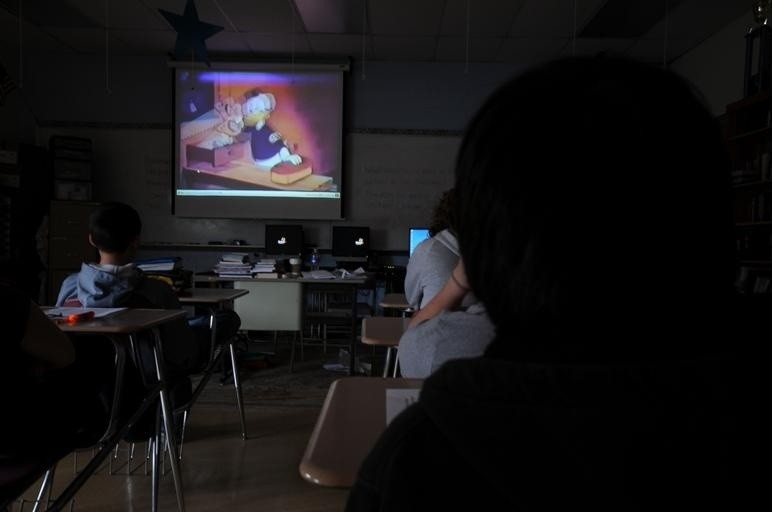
68, 311, 95, 323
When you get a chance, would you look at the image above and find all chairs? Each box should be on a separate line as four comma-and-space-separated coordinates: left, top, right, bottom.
60, 296, 192, 478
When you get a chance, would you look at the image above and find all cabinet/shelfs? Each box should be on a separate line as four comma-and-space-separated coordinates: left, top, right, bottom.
49, 199, 98, 272
720, 88, 771, 297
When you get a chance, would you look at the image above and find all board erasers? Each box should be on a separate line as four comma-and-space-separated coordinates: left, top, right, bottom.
208, 242, 223, 245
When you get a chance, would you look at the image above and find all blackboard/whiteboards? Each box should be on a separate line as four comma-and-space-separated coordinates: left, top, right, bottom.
34, 116, 469, 256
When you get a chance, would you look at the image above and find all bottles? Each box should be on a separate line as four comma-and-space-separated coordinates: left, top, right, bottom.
309, 247, 321, 271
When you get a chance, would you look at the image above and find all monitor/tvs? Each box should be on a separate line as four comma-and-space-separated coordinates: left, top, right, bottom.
409, 227, 432, 259
332, 226, 369, 262
264, 224, 304, 259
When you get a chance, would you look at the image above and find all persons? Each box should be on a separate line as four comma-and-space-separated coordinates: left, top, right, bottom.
0, 273, 76, 471
346, 53, 772, 511
55, 202, 241, 446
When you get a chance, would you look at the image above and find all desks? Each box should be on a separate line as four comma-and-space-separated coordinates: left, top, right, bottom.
42, 304, 194, 511
176, 285, 254, 459
376, 291, 418, 318
298, 374, 432, 488
359, 316, 413, 377
195, 273, 369, 373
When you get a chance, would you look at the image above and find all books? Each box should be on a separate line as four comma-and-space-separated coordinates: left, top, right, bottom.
213, 252, 284, 279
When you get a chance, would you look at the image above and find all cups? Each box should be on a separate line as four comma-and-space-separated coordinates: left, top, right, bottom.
289, 255, 303, 275
